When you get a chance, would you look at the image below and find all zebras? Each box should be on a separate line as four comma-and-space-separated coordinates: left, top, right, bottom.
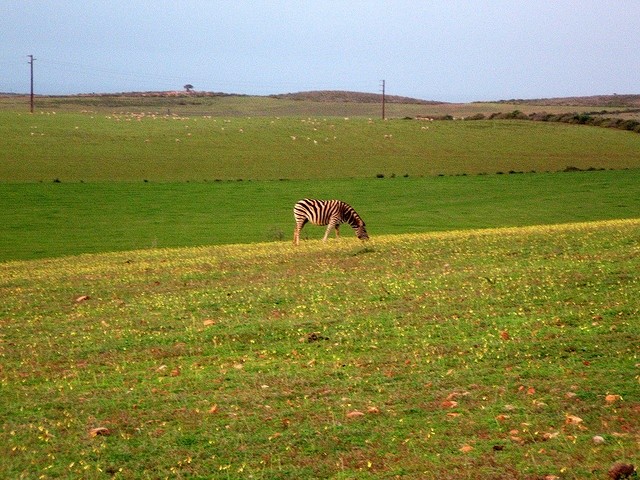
291, 197, 370, 244
